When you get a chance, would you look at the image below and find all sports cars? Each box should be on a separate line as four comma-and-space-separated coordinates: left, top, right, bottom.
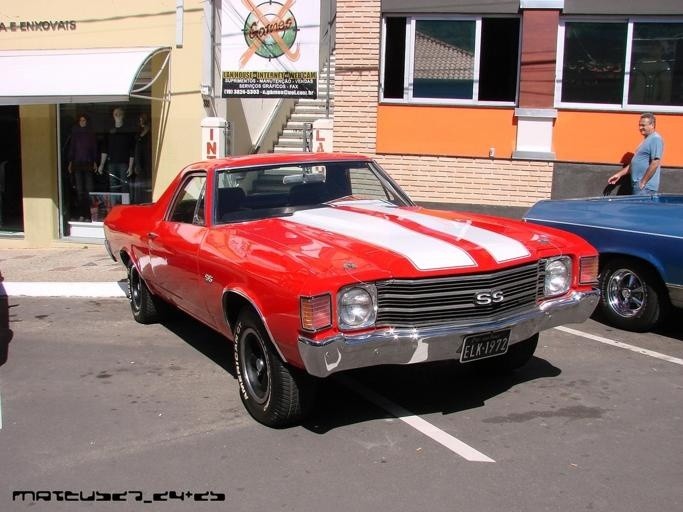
101, 155, 606, 431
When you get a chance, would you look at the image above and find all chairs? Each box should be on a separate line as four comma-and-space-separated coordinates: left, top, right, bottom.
288, 182, 333, 206
216, 186, 254, 215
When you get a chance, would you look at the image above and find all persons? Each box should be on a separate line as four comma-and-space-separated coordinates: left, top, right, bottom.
131, 112, 150, 202
606, 113, 664, 196
66, 113, 99, 222
97, 107, 135, 192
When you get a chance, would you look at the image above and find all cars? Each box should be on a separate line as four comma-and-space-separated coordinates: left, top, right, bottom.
523, 192, 683, 334
